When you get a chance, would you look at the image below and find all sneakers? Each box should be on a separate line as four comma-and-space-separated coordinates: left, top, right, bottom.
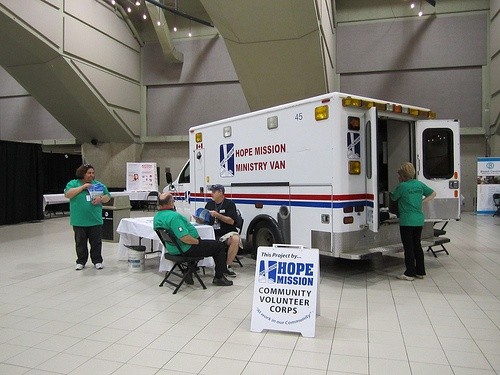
223, 264, 237, 277
183, 266, 201, 273
95, 263, 104, 269
75, 264, 85, 270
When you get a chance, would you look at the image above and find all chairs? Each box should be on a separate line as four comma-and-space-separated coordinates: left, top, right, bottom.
143, 191, 158, 212
427, 222, 450, 258
233, 219, 244, 267
152, 228, 207, 295
493, 194, 500, 217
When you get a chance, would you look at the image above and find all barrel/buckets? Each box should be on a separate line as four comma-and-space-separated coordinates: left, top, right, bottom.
128, 248, 145, 273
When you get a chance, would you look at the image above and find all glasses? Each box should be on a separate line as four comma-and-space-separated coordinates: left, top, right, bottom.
212, 185, 220, 191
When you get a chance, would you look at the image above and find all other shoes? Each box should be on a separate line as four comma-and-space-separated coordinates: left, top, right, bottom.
415, 274, 424, 279
183, 268, 194, 285
213, 276, 233, 286
396, 274, 415, 281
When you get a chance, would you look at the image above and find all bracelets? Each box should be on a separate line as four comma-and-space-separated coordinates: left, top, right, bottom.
99, 196, 102, 201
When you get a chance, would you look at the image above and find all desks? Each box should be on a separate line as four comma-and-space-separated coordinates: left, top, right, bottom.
43, 191, 161, 219
116, 217, 215, 277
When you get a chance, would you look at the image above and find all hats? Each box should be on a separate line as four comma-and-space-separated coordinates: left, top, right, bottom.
208, 183, 225, 193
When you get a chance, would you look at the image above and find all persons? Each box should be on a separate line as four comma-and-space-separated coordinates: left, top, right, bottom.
153, 193, 233, 286
391, 162, 436, 280
204, 184, 240, 276
63, 164, 111, 270
